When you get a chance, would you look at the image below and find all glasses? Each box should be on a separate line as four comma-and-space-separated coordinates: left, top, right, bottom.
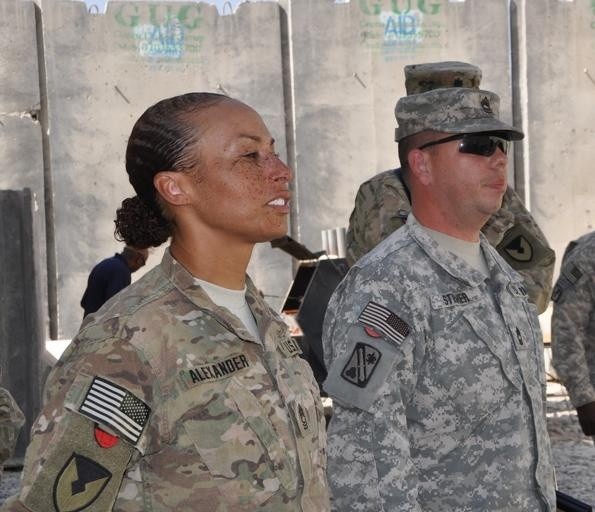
418, 132, 507, 156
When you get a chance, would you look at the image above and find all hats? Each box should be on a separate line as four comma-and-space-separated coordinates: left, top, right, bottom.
394, 87, 524, 140
405, 61, 482, 95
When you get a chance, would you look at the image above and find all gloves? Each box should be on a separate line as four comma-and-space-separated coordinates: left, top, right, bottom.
576, 401, 594, 439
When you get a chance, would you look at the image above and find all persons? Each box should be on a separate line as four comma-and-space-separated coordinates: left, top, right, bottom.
551, 231, 595, 447
0, 92, 331, 512
81, 245, 148, 323
0, 387, 26, 483
346, 62, 555, 317
321, 86, 557, 512
